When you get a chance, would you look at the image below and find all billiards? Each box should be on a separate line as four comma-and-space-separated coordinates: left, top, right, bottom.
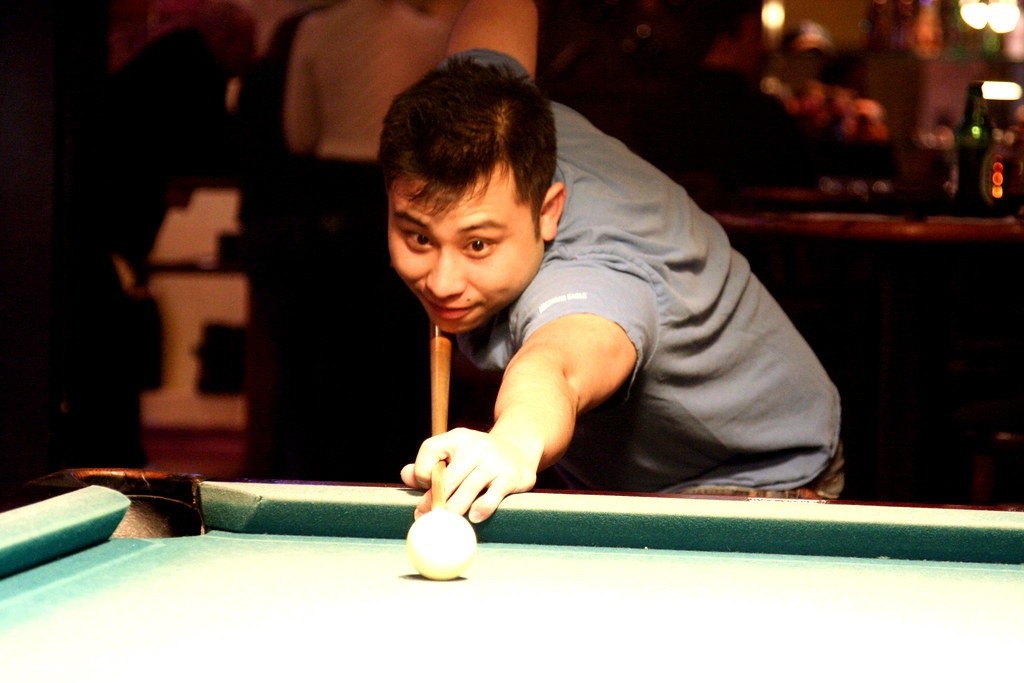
406, 508, 478, 578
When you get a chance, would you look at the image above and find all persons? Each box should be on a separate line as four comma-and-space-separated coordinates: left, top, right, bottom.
110, 1, 469, 482
376, 1, 846, 524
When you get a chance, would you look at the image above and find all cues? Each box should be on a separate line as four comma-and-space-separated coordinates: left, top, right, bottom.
427, 316, 453, 505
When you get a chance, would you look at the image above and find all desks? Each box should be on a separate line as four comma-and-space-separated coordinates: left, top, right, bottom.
0, 460, 1024, 674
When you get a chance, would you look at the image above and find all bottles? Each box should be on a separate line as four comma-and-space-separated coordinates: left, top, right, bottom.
952, 81, 996, 217
910, 0, 943, 61
